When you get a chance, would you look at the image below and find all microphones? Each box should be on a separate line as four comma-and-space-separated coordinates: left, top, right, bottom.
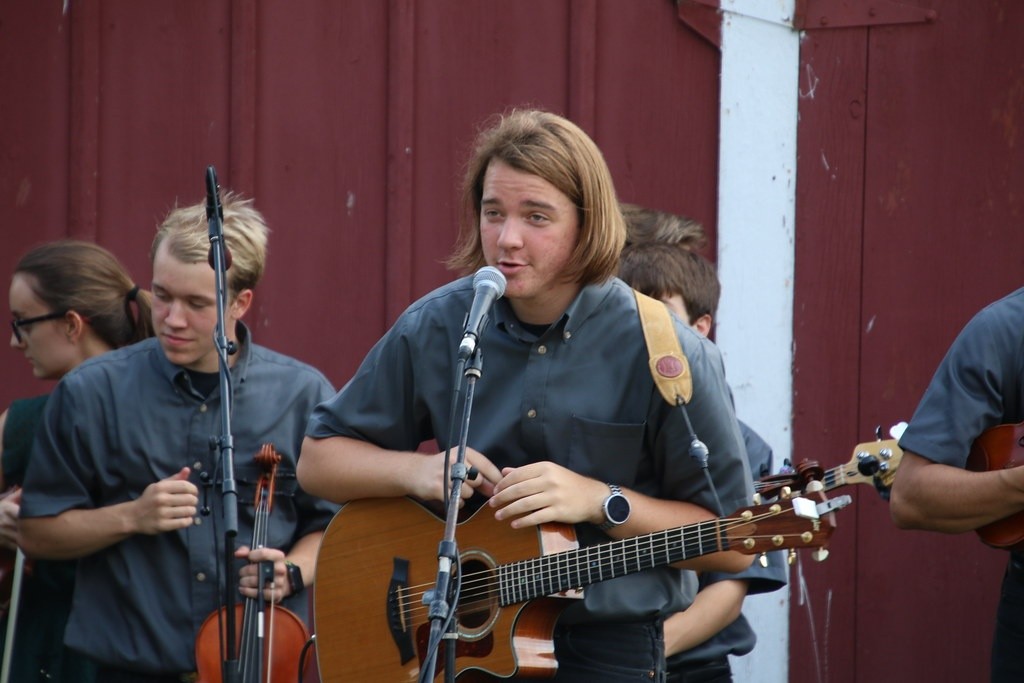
204, 165, 232, 271
457, 265, 507, 361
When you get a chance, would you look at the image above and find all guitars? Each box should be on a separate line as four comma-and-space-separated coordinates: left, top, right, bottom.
310, 477, 852, 683
956, 421, 1024, 551
747, 417, 913, 505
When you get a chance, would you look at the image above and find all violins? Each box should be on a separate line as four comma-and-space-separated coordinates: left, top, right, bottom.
190, 442, 309, 682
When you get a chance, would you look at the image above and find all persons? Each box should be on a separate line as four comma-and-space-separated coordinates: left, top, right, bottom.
616, 242, 788, 681
19, 192, 340, 683
890, 287, 1023, 682
294, 109, 752, 683
0, 243, 149, 680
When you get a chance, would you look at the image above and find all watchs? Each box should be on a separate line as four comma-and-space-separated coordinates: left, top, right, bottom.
284, 560, 304, 595
599, 481, 632, 533
